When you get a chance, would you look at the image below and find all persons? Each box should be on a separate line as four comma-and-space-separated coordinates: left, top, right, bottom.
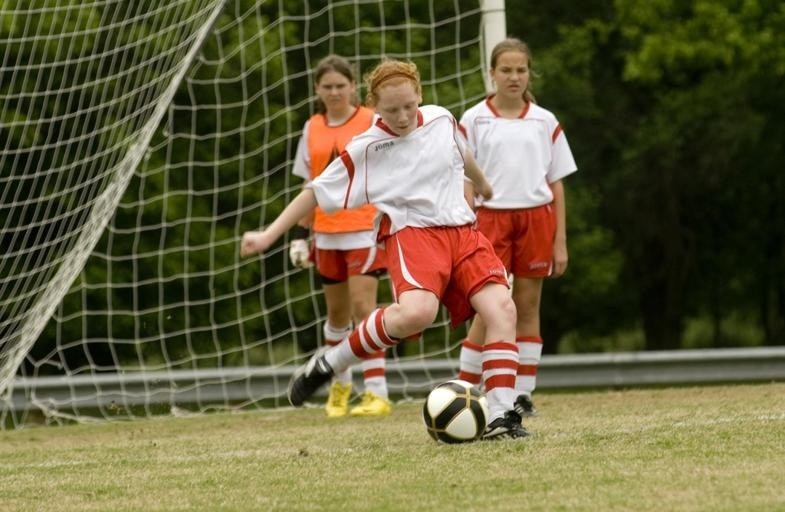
239, 54, 532, 440
287, 52, 393, 419
457, 36, 578, 419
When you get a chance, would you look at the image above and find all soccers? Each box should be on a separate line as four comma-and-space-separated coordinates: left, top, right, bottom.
422, 380, 489, 443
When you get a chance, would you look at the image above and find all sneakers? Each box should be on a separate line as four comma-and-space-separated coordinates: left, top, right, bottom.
350, 391, 392, 416
288, 346, 334, 409
482, 411, 531, 439
326, 381, 352, 416
514, 395, 537, 416
